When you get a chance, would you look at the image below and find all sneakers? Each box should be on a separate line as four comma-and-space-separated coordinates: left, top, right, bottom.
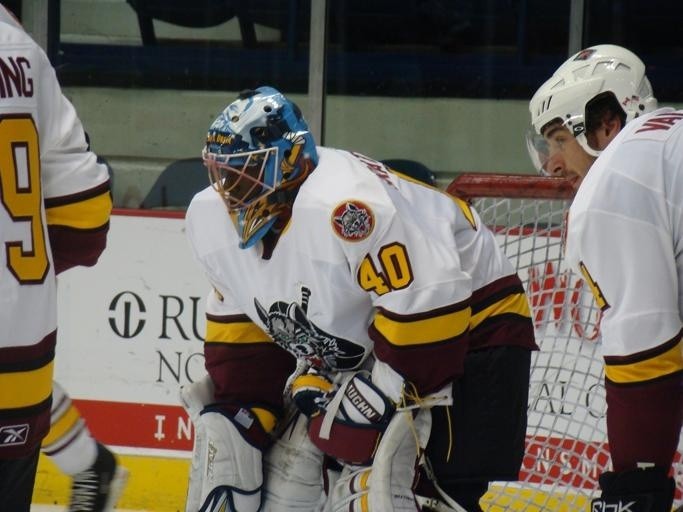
70, 442, 116, 512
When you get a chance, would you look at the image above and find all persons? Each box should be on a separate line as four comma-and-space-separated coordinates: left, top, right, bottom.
38, 379, 118, 511
182, 88, 541, 512
0, 4, 114, 512
521, 42, 683, 512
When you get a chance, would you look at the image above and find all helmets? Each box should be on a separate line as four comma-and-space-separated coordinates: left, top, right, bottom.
202, 84, 317, 251
528, 44, 659, 178
